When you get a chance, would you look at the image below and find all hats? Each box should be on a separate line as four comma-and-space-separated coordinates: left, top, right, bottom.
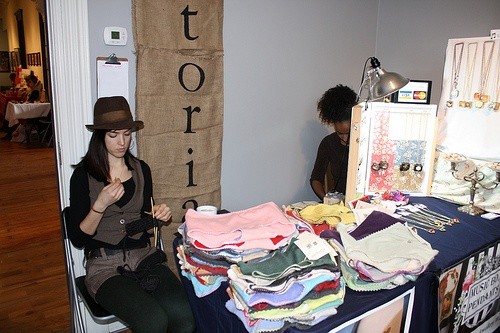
85, 95, 145, 133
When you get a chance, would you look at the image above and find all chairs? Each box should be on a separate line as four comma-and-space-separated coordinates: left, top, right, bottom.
61, 206, 180, 333
39, 119, 54, 146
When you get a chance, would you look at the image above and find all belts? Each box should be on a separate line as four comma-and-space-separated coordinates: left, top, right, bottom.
86, 237, 150, 258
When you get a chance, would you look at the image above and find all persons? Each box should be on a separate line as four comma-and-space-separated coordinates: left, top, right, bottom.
0, 75, 43, 141
9, 73, 17, 92
69, 96, 201, 333
310, 85, 362, 203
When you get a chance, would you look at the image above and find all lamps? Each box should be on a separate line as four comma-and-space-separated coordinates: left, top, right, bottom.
357, 56, 412, 104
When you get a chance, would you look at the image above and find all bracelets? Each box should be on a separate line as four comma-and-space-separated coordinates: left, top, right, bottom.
91, 207, 106, 214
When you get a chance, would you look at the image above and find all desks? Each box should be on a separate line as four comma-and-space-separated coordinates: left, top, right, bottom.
5, 101, 52, 144
173, 195, 500, 333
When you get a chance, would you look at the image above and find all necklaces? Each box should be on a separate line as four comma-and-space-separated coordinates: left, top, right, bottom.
445, 40, 500, 112
371, 113, 423, 172
393, 202, 461, 234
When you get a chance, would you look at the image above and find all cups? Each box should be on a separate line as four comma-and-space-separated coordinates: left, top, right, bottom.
197, 206, 217, 215
